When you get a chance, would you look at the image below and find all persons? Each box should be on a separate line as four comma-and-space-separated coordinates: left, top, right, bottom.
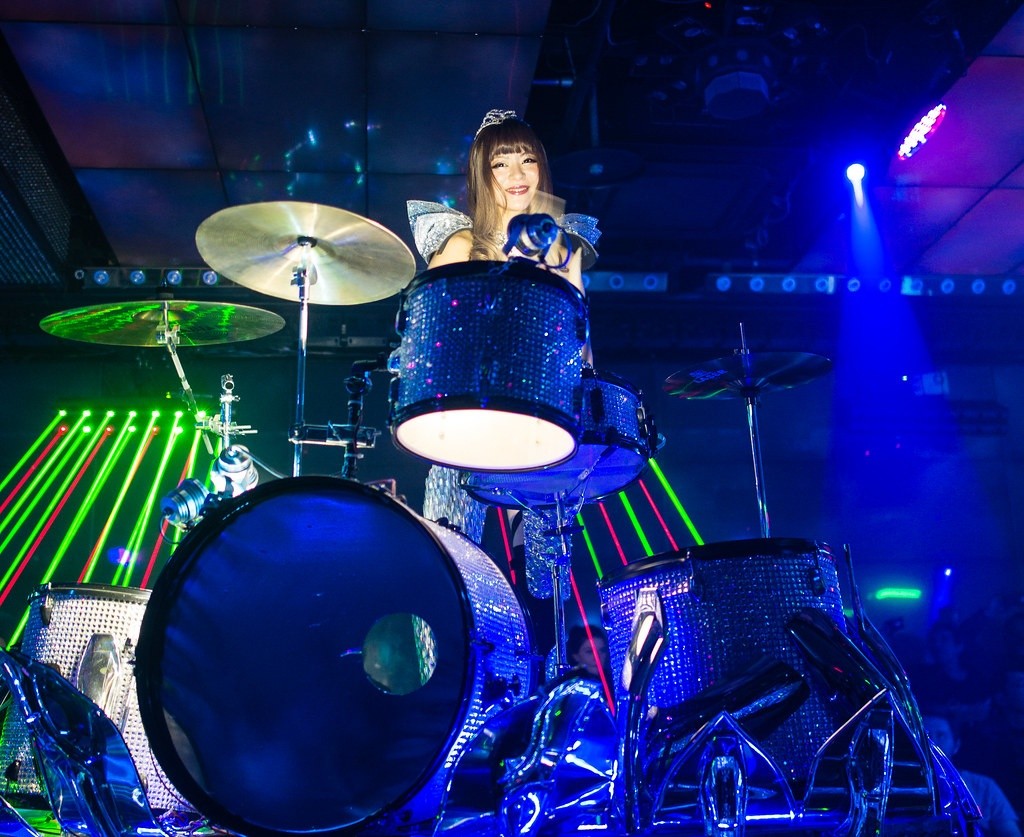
423, 109, 594, 600
882, 599, 1024, 837
565, 623, 607, 674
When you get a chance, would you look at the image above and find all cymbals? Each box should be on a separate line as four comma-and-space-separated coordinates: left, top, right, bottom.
36, 299, 286, 348
194, 200, 416, 306
662, 348, 838, 400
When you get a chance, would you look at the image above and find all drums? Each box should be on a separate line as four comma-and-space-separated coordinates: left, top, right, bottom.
459, 364, 652, 512
595, 533, 853, 743
133, 473, 543, 837
1, 583, 210, 835
386, 258, 591, 474
